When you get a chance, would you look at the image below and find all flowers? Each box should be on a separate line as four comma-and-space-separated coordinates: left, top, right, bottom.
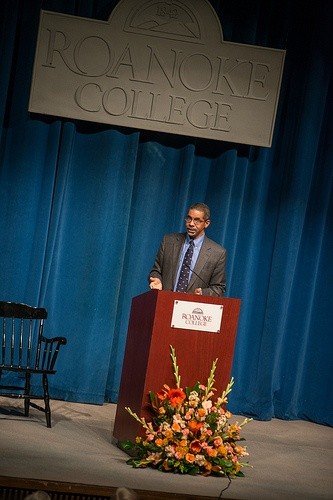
116, 344, 254, 480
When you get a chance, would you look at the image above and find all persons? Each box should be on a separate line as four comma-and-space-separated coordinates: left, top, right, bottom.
148, 203, 226, 297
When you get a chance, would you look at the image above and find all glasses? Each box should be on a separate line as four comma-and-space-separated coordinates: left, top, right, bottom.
184, 216, 206, 225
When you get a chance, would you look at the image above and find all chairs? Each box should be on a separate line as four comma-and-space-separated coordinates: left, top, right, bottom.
0, 300, 67, 428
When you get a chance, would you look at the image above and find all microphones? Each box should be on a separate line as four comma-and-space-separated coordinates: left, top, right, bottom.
184, 259, 221, 297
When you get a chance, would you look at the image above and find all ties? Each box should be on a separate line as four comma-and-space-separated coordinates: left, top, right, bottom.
176, 241, 194, 293
